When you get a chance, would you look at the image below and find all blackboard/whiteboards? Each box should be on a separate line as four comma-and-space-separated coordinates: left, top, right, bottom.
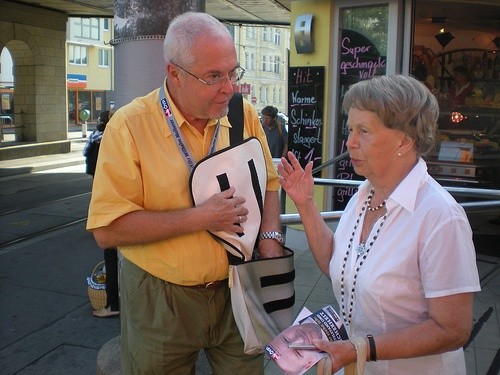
286, 66, 325, 178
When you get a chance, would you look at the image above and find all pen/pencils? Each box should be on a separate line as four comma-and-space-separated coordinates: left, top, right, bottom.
289, 344, 318, 350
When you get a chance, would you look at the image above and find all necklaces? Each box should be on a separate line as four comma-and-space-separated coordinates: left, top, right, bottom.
340, 194, 389, 326
356, 212, 383, 254
367, 187, 387, 211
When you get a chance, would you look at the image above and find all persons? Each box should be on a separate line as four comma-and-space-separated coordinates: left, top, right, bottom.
85, 11, 285, 375
260, 105, 288, 158
91, 246, 121, 318
277, 73, 482, 375
82, 110, 110, 178
411, 52, 439, 91
448, 64, 476, 109
267, 322, 322, 375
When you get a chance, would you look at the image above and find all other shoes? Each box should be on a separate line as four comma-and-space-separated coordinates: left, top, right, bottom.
93, 306, 120, 317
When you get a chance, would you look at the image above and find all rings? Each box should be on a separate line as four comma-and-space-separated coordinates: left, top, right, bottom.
239, 216, 242, 222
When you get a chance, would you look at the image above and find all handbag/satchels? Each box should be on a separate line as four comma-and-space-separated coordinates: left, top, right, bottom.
85, 141, 100, 175
188, 136, 296, 357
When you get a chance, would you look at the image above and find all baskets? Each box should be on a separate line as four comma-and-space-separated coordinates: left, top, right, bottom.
86, 260, 108, 309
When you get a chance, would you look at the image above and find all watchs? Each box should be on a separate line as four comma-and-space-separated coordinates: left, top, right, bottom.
259, 231, 285, 246
366, 334, 377, 363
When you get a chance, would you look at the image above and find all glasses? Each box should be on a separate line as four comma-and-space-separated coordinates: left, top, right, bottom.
169, 59, 245, 89
